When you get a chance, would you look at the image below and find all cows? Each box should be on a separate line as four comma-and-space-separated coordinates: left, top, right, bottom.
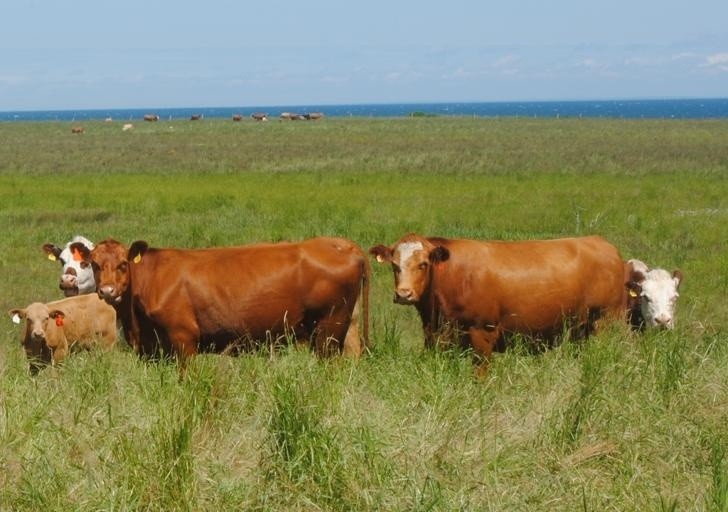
367, 230, 630, 386
41, 235, 98, 298
5, 291, 120, 380
69, 235, 372, 385
590, 257, 683, 334
